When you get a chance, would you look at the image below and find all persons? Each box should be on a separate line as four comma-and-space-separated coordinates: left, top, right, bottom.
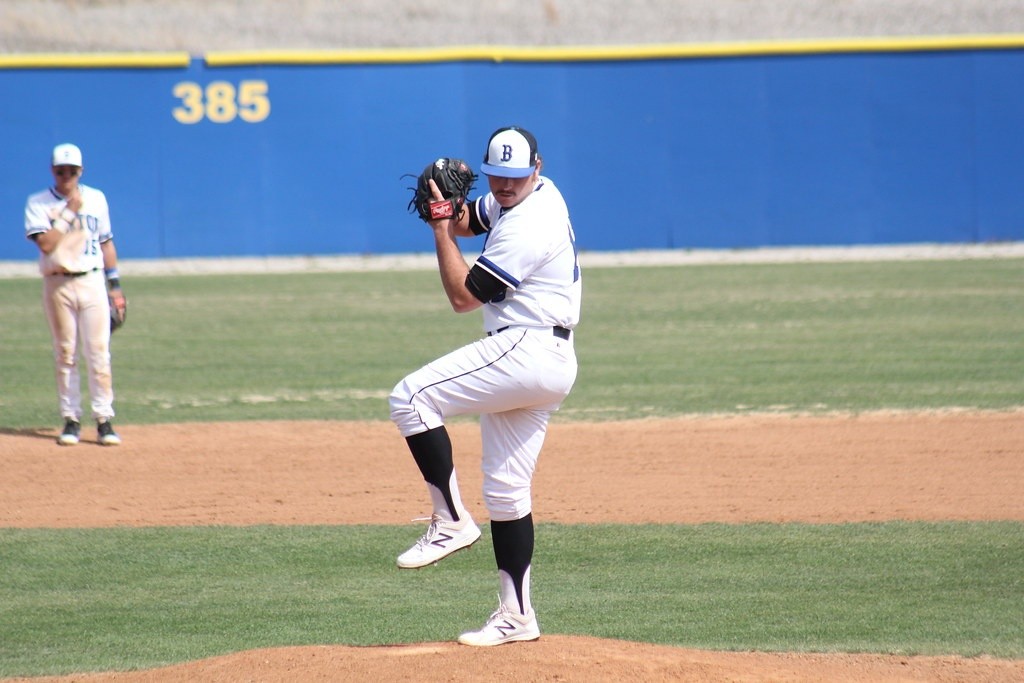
389, 126, 581, 647
25, 143, 126, 445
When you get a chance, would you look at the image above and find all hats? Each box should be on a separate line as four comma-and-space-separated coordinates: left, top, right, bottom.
480, 126, 538, 178
53, 143, 82, 167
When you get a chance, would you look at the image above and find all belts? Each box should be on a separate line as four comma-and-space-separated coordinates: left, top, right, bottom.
487, 326, 571, 340
52, 268, 97, 276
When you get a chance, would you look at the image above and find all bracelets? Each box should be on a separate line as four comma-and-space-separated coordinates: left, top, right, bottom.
104, 267, 119, 280
53, 217, 72, 234
106, 278, 121, 291
60, 207, 75, 223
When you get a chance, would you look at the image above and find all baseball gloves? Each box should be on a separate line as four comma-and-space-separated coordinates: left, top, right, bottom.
109, 294, 126, 332
416, 158, 474, 224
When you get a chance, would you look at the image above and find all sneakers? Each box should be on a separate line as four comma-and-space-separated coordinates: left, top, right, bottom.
58, 420, 80, 445
396, 510, 482, 569
458, 591, 540, 647
98, 418, 121, 445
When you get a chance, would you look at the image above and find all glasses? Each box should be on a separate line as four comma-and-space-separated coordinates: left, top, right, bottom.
56, 169, 78, 176
